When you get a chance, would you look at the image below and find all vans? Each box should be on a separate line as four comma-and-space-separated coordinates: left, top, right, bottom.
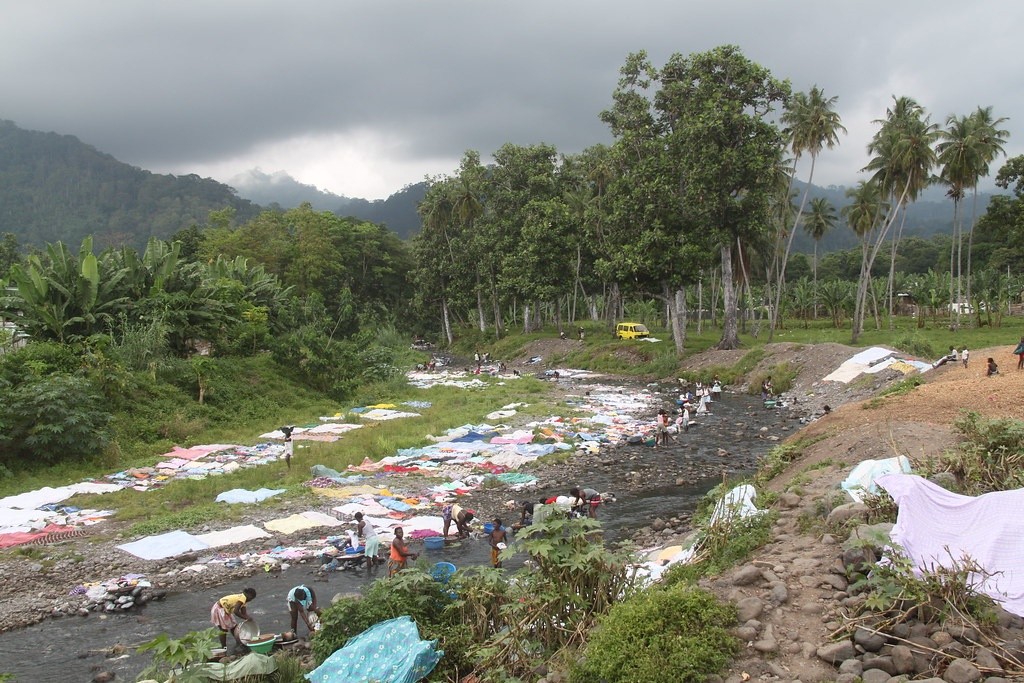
616, 322, 650, 341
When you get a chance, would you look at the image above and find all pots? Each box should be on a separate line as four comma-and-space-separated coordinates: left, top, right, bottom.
207, 647, 228, 662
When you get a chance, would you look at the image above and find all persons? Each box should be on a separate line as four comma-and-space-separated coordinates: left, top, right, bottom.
442, 505, 473, 538
285, 584, 325, 635
518, 488, 603, 525
389, 527, 409, 578
655, 374, 722, 445
416, 328, 590, 379
761, 376, 833, 418
211, 587, 257, 649
283, 432, 293, 469
488, 518, 508, 568
913, 335, 1024, 387
349, 512, 379, 574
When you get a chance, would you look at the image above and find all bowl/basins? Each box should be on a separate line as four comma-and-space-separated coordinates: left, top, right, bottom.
483, 523, 505, 534
423, 538, 445, 549
429, 561, 456, 584
345, 546, 365, 554
645, 439, 656, 447
234, 620, 276, 655
